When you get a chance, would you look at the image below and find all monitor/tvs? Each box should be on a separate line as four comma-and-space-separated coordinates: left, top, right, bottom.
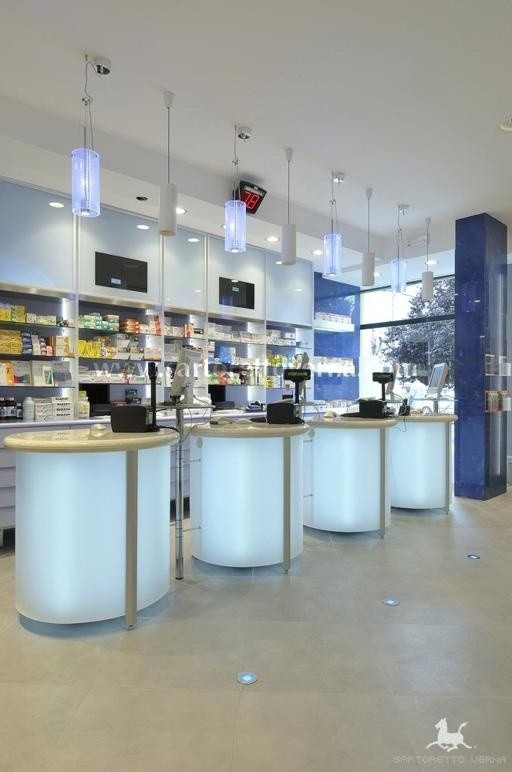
298, 352, 310, 402
427, 363, 448, 399
385, 364, 399, 401
170, 348, 202, 406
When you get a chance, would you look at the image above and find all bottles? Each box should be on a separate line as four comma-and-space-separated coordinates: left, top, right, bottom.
0, 397, 23, 420
80, 397, 90, 418
22, 397, 34, 422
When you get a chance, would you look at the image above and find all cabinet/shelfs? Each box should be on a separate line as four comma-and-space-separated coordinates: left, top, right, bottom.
0, 281, 314, 425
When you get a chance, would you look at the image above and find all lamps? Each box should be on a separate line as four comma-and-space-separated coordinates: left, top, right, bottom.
72, 53, 433, 301
